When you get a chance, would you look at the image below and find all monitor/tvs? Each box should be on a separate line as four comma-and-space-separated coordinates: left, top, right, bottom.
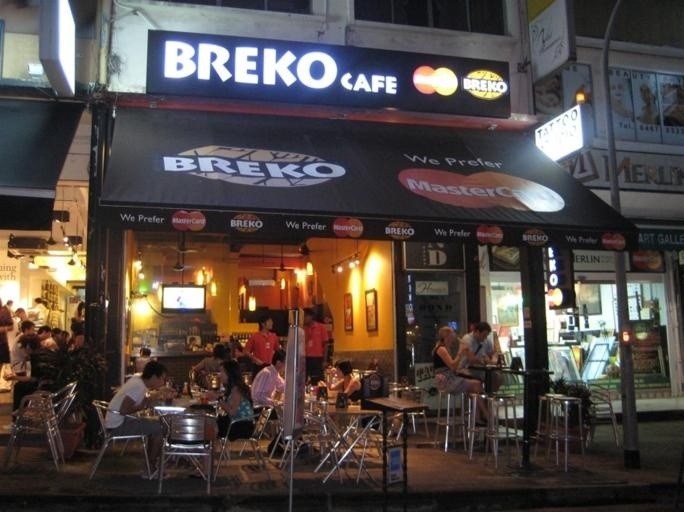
160, 283, 206, 314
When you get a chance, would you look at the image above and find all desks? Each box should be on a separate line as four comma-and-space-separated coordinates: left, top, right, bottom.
474, 360, 554, 471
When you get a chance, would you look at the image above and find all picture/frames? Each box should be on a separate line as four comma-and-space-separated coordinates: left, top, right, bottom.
364, 290, 379, 334
342, 294, 353, 332
567, 283, 603, 315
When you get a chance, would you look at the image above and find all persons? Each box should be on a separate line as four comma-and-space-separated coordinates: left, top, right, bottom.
1, 295, 114, 430
536, 72, 658, 126
103, 340, 256, 464
431, 325, 490, 428
244, 307, 364, 458
458, 321, 504, 422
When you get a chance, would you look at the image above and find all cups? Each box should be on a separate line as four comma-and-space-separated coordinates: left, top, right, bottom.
200, 393, 211, 405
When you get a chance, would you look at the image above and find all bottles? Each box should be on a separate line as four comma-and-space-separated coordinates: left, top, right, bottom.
159, 327, 217, 336
181, 383, 189, 397
231, 332, 252, 347
164, 390, 173, 406
582, 304, 590, 328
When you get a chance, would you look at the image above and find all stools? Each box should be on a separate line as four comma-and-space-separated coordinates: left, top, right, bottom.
434, 376, 619, 471
